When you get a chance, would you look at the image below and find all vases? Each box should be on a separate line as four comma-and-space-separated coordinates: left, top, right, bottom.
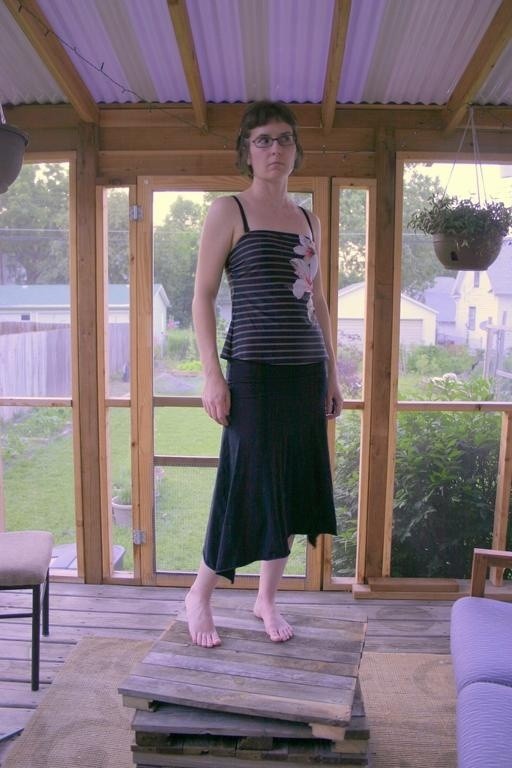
0, 123, 29, 194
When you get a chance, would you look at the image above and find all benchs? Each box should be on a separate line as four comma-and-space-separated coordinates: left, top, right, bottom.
450, 549, 512, 768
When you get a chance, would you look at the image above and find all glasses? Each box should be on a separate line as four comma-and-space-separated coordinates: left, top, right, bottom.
238, 132, 298, 150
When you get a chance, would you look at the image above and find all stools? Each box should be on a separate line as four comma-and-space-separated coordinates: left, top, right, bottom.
1, 531, 55, 692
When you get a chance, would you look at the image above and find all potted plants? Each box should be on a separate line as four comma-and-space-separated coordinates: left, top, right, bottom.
112, 466, 131, 529
408, 192, 512, 271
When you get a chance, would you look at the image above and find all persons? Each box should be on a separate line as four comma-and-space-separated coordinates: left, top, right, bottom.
182, 98, 346, 651
